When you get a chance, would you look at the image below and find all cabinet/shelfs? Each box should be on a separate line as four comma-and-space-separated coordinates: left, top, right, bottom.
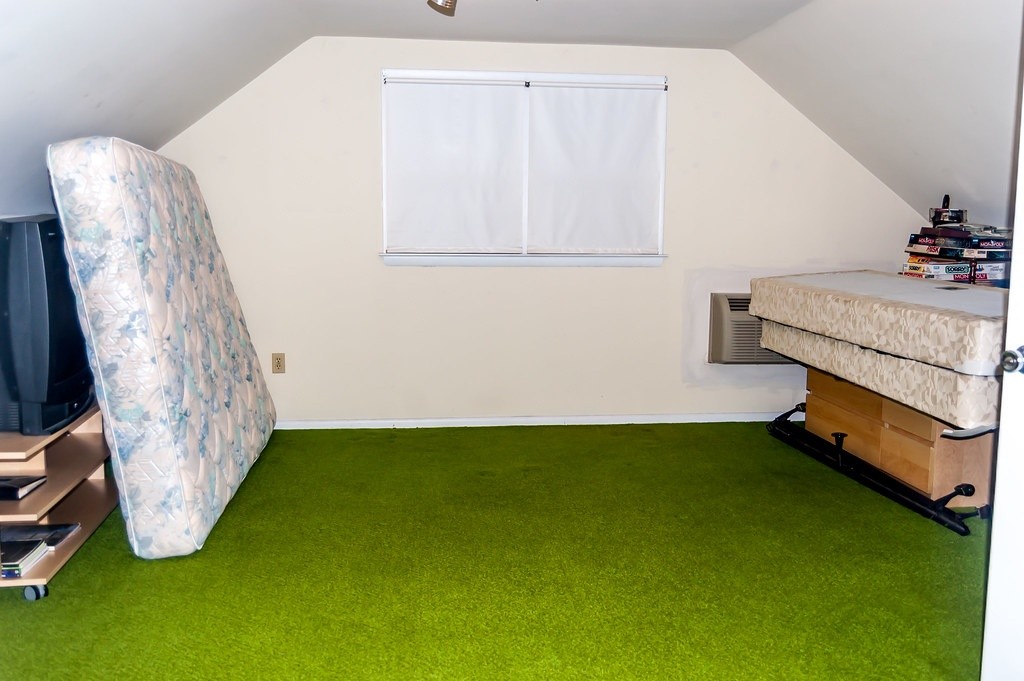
0, 405, 119, 600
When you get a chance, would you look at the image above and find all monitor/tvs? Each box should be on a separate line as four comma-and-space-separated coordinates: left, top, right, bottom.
2, 215, 95, 437
